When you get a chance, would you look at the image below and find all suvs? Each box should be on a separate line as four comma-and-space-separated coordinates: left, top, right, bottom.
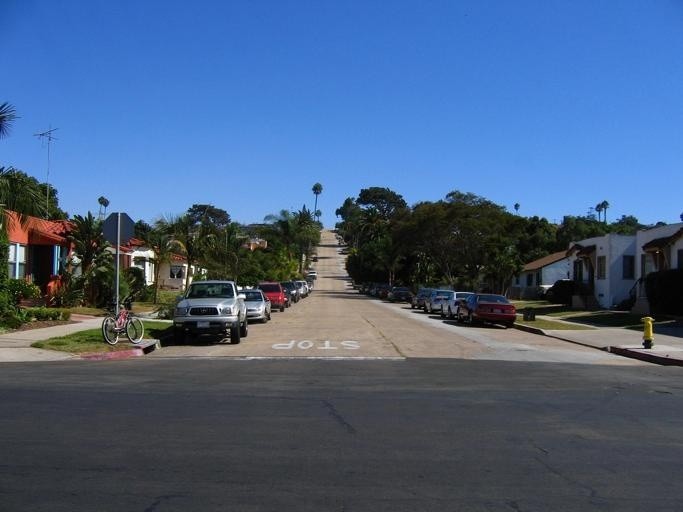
172, 280, 250, 346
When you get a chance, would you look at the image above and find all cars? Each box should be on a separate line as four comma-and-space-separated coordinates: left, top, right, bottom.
358, 281, 519, 329
237, 270, 318, 324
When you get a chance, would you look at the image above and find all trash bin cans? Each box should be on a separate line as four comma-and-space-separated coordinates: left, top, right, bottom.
523, 307, 536, 321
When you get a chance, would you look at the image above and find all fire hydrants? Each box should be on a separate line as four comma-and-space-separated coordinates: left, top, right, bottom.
640, 317, 656, 349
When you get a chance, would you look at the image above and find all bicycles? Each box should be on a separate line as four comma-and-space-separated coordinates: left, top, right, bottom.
101, 296, 145, 346
